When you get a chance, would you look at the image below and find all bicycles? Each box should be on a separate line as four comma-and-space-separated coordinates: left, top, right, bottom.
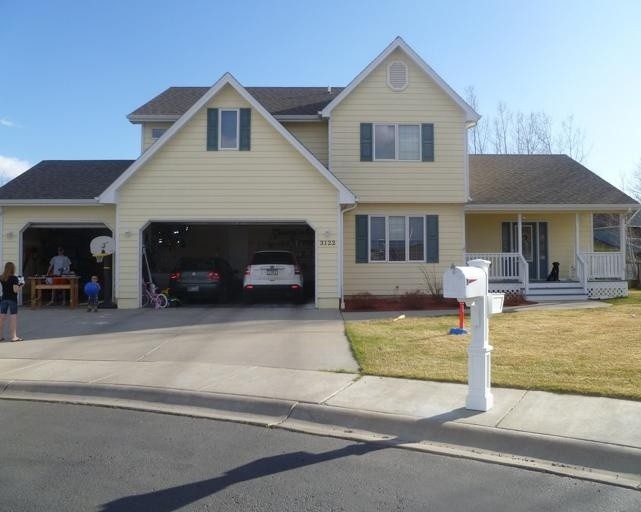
142, 280, 168, 309
161, 287, 181, 307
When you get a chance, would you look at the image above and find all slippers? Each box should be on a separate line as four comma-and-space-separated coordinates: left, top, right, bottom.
11, 336, 23, 342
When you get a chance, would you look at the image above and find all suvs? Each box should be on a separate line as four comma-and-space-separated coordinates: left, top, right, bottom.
242, 251, 304, 303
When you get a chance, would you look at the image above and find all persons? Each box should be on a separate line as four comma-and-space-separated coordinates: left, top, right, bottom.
84, 274, 101, 312
46, 246, 72, 306
0, 262, 24, 342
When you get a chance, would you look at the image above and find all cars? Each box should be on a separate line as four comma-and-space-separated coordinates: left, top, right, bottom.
169, 257, 241, 305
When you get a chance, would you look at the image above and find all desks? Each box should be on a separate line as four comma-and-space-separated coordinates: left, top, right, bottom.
28, 273, 82, 312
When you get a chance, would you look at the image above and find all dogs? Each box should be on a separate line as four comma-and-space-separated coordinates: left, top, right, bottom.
545, 262, 560, 281
